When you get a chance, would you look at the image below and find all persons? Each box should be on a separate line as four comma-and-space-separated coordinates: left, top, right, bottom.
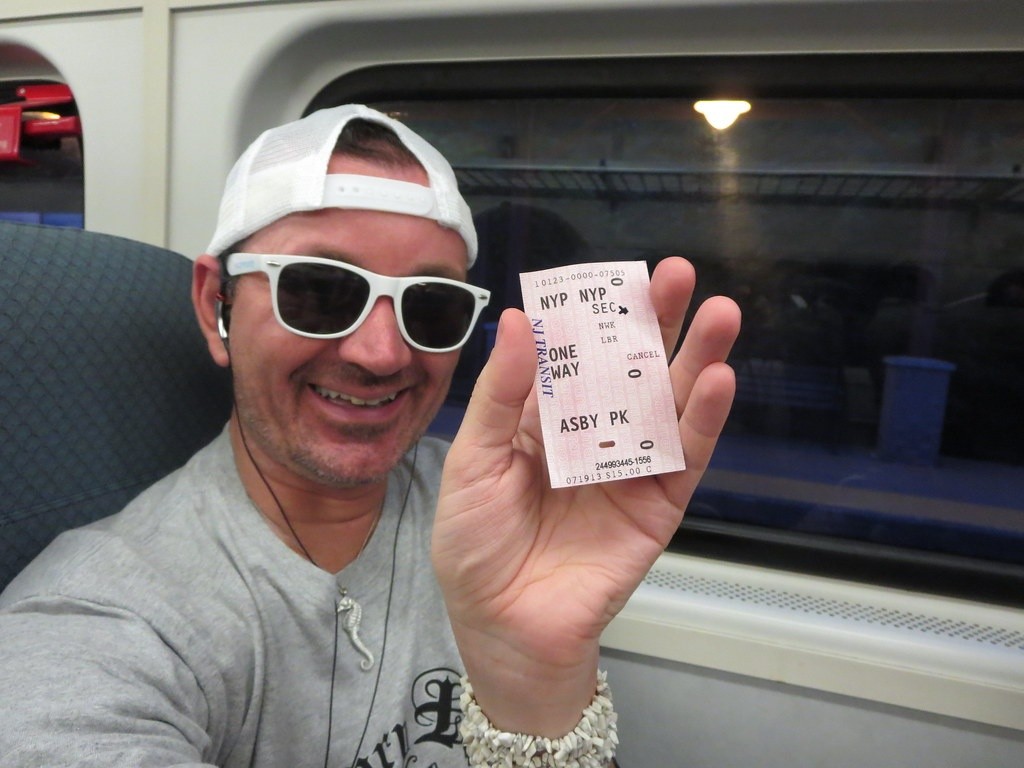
0, 103, 740, 768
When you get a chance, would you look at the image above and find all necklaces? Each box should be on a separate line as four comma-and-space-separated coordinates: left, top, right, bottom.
246, 482, 385, 672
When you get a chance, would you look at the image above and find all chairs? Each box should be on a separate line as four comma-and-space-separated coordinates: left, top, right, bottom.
0, 219, 236, 598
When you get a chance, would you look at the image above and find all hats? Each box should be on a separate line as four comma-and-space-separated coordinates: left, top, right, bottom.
206, 103, 479, 272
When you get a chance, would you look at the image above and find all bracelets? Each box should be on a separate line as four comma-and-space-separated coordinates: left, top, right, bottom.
458, 669, 619, 768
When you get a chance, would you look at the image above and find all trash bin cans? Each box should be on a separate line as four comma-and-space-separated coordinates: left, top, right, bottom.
876, 354, 959, 466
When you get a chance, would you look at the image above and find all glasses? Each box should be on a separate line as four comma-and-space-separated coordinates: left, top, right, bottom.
224, 251, 492, 354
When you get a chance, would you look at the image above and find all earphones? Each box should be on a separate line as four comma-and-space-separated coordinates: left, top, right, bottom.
215, 301, 230, 342
